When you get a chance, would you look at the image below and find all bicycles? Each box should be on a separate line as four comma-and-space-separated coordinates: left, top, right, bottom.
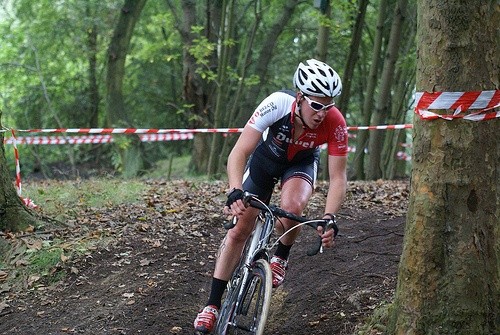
214, 189, 339, 335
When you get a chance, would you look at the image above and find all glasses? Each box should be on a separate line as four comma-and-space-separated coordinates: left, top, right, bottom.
300, 90, 335, 112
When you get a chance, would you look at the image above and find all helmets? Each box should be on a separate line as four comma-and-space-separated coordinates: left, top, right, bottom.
293, 59, 342, 97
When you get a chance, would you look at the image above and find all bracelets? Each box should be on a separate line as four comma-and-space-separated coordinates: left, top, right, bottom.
323, 213, 337, 224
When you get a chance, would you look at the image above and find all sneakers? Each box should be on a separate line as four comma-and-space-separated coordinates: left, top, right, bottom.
269, 255, 288, 288
193, 305, 220, 333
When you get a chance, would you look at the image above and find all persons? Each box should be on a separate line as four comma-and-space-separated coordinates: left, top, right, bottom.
193, 59, 348, 334
